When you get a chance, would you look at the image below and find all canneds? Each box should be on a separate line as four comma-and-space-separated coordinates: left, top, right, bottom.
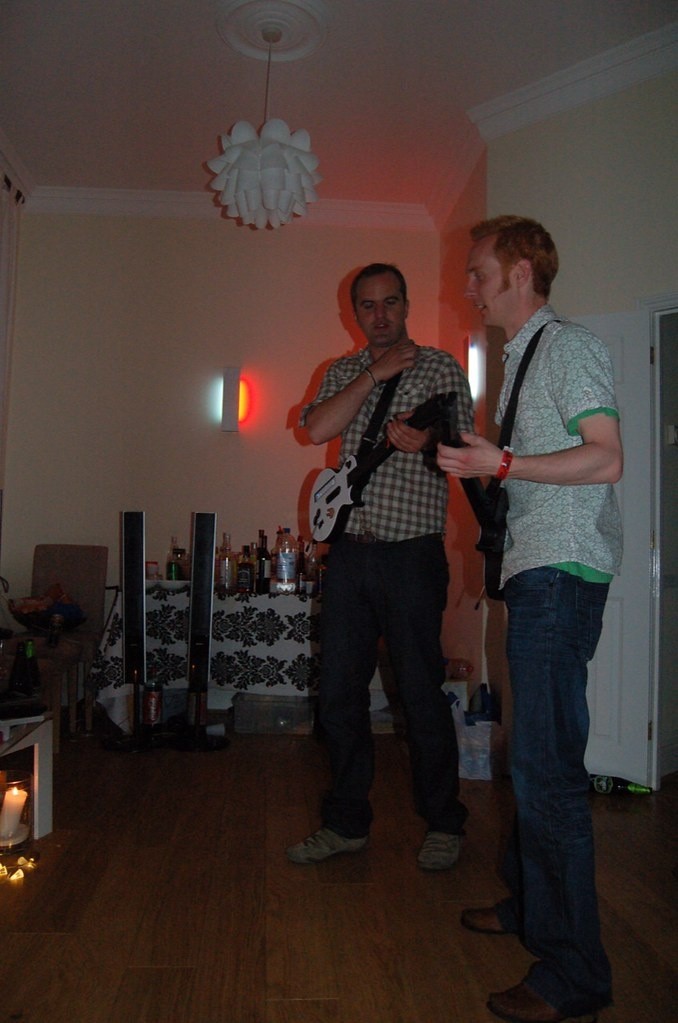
166, 560, 178, 580
49, 613, 65, 630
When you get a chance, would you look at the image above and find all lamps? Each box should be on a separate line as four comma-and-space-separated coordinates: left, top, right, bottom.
205, 0, 324, 228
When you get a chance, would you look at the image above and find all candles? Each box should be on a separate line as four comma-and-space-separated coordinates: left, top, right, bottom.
0, 787, 27, 841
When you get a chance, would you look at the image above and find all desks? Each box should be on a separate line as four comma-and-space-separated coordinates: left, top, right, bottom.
0, 687, 54, 839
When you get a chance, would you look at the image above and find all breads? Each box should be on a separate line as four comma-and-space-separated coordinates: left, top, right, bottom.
13, 584, 74, 613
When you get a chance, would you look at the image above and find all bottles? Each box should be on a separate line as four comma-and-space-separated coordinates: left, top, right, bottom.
270, 527, 304, 593
0, 639, 11, 692
141, 667, 163, 753
307, 539, 320, 580
238, 543, 258, 593
10, 642, 32, 707
590, 775, 653, 795
168, 537, 178, 561
443, 656, 473, 680
256, 530, 272, 594
215, 532, 238, 589
26, 639, 42, 707
47, 615, 66, 664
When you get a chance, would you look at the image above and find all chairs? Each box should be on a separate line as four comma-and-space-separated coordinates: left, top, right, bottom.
0, 544, 119, 753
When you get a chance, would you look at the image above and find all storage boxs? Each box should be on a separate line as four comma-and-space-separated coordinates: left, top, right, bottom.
232, 693, 316, 735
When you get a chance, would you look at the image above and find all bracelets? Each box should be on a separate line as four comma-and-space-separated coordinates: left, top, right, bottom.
494, 451, 513, 482
364, 367, 377, 388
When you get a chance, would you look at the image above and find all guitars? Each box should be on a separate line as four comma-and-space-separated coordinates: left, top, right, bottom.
444, 389, 509, 602
307, 388, 461, 543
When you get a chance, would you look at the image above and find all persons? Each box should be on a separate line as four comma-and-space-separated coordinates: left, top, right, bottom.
437, 215, 623, 1022
287, 262, 473, 874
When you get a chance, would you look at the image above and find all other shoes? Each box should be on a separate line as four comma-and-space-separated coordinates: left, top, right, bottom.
460, 906, 503, 936
286, 828, 368, 864
417, 831, 460, 870
487, 982, 566, 1023
